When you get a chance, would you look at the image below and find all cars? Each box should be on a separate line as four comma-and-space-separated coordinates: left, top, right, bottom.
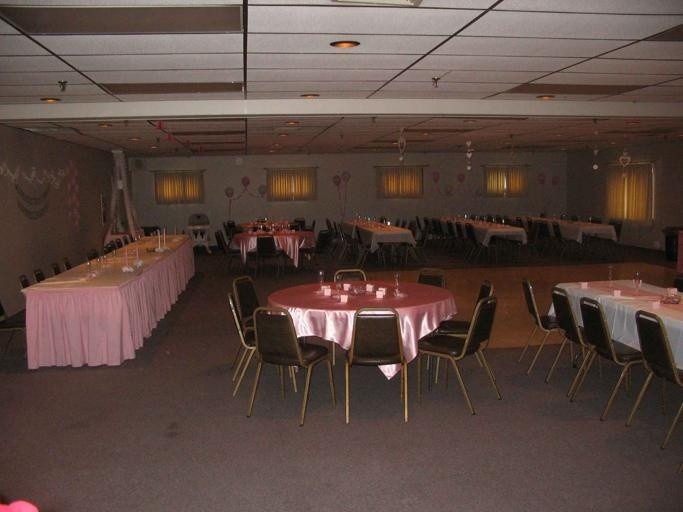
321, 283, 386, 303
580, 282, 678, 309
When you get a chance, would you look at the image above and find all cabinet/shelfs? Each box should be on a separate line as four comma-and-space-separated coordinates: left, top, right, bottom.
364, 215, 391, 226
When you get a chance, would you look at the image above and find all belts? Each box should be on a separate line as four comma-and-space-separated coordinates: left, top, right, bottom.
631, 271, 641, 296
607, 262, 616, 289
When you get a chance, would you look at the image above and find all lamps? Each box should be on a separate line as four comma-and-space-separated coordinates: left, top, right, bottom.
625, 311, 683, 449
416, 269, 446, 290
416, 297, 501, 416
427, 281, 495, 383
545, 285, 630, 397
570, 298, 666, 421
247, 308, 337, 425
517, 278, 586, 379
84, 235, 136, 261
227, 291, 298, 397
232, 276, 292, 379
0, 299, 26, 362
345, 307, 409, 425
17, 259, 71, 286
334, 269, 367, 282
